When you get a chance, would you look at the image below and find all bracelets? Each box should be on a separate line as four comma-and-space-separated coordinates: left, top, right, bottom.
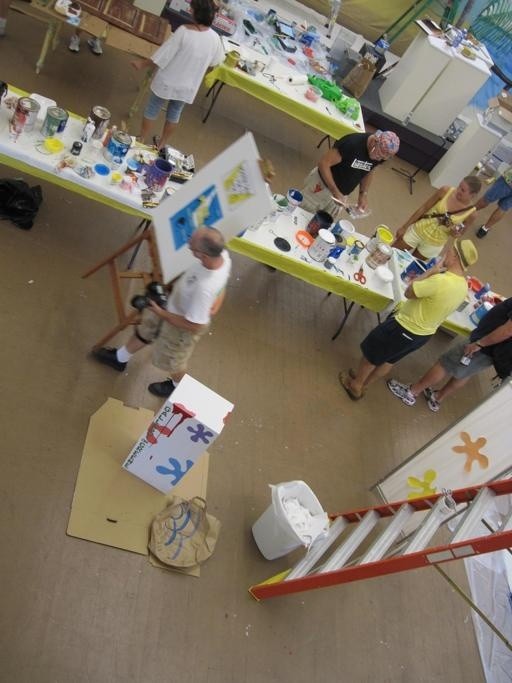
357, 190, 370, 196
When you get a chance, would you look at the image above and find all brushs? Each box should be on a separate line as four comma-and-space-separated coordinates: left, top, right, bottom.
331, 195, 352, 214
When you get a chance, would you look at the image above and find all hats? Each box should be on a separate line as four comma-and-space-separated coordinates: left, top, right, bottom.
376, 129, 400, 160
454, 238, 478, 272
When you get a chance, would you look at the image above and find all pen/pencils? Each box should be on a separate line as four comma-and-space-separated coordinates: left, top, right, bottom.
326, 106, 332, 116
229, 40, 240, 47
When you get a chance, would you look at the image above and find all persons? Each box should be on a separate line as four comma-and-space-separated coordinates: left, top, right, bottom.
338, 238, 478, 403
455, 140, 511, 241
299, 126, 401, 220
391, 174, 480, 270
129, 0, 227, 152
387, 273, 512, 412
70, 28, 107, 58
90, 224, 233, 401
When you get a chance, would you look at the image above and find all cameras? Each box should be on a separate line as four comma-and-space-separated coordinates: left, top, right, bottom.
130, 281, 167, 310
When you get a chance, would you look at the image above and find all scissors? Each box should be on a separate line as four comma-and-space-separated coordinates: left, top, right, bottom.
354, 263, 365, 283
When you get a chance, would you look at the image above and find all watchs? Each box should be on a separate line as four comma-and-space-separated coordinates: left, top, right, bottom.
475, 340, 484, 350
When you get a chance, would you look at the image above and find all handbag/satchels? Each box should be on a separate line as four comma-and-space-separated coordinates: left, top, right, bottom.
149, 495, 221, 570
341, 58, 376, 100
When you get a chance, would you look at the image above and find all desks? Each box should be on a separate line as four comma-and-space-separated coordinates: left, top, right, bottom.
366, 247, 506, 340
203, 36, 366, 152
1, 1, 175, 119
229, 196, 394, 339
1, 81, 190, 277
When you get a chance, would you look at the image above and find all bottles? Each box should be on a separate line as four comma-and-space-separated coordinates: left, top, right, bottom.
451, 28, 468, 49
474, 281, 491, 300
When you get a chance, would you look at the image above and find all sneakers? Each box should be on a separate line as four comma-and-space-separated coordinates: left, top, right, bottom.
149, 380, 175, 397
91, 347, 127, 372
477, 224, 490, 237
340, 368, 440, 412
136, 135, 160, 146
69, 35, 102, 54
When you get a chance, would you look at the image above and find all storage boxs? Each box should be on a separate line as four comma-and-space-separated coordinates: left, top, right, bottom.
118, 370, 235, 495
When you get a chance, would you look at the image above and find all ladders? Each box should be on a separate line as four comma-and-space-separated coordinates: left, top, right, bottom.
247, 478, 512, 601
